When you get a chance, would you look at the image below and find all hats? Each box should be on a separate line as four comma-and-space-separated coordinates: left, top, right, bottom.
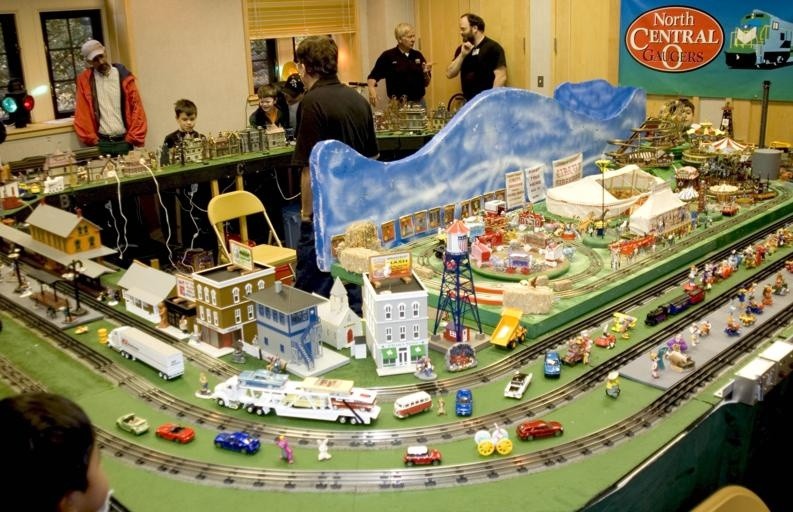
82, 40, 105, 61
282, 74, 304, 98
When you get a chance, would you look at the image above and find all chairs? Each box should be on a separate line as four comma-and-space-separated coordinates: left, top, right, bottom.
207, 190, 297, 284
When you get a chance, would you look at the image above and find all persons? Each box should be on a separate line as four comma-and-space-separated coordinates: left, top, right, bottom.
249, 84, 282, 130
160, 99, 206, 167
367, 22, 437, 114
668, 98, 695, 130
281, 73, 305, 138
1, 393, 109, 512
73, 39, 147, 158
290, 35, 380, 317
446, 13, 507, 101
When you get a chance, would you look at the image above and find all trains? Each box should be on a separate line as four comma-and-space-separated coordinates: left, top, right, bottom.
723, 9, 793, 68
